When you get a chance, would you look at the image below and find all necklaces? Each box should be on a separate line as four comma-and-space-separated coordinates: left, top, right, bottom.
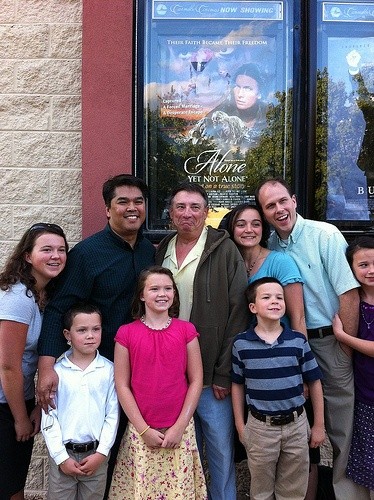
141, 314, 171, 330
247, 247, 262, 278
361, 300, 374, 329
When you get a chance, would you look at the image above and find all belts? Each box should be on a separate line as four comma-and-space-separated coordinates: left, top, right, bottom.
306, 326, 334, 340
251, 406, 303, 426
65, 440, 100, 453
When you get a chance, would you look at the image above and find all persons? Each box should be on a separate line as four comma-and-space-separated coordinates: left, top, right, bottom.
346, 49, 374, 220
0, 174, 374, 500
205, 64, 269, 129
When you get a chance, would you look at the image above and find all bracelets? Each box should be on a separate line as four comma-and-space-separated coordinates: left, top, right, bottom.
139, 426, 150, 436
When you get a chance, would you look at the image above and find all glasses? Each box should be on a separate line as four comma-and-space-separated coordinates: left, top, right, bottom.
29, 223, 64, 236
